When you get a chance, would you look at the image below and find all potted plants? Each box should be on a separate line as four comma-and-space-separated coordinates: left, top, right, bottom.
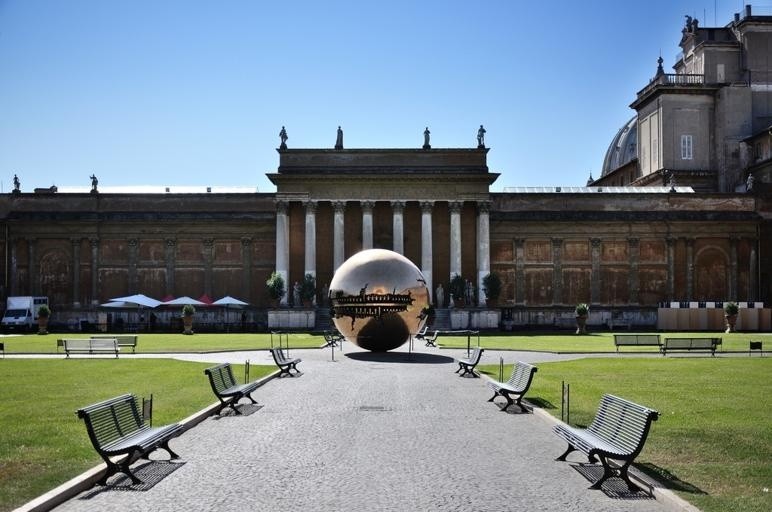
296, 276, 317, 308
724, 304, 739, 334
482, 273, 502, 308
37, 305, 51, 333
574, 303, 591, 335
182, 304, 196, 335
267, 273, 283, 310
447, 278, 467, 309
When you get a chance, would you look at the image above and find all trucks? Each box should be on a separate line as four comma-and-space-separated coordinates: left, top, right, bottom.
1, 296, 49, 334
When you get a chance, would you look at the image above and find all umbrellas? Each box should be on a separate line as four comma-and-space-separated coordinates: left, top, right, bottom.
100, 293, 250, 333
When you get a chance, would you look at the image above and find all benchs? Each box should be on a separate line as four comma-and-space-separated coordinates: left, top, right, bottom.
205, 362, 258, 415
614, 334, 662, 353
90, 336, 137, 354
415, 326, 429, 340
75, 393, 183, 486
425, 330, 439, 347
63, 340, 119, 359
323, 330, 338, 348
455, 347, 484, 378
480, 361, 537, 412
663, 339, 717, 355
552, 392, 660, 492
270, 345, 303, 377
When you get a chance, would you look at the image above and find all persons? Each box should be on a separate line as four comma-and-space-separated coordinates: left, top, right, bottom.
424, 127, 431, 145
13, 174, 21, 190
278, 126, 289, 144
89, 174, 99, 190
335, 125, 344, 146
478, 125, 487, 145
